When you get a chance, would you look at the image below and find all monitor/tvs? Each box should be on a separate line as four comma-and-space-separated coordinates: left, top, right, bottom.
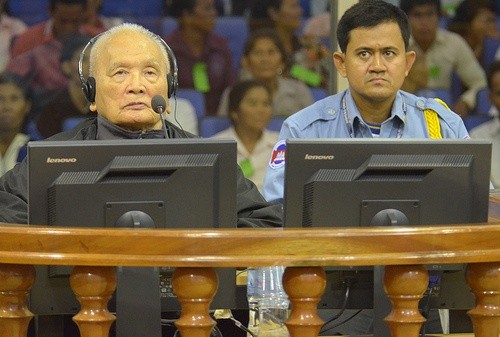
27, 138, 238, 337
282, 138, 493, 337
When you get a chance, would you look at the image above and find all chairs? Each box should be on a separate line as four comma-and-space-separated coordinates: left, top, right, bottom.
0, 0, 500, 137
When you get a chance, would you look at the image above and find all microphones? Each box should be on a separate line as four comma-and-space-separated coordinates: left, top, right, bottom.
151, 95, 168, 139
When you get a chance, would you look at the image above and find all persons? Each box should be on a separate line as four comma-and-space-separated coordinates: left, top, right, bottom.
247, 0, 494, 337
0, 23, 283, 337
0, 0, 500, 194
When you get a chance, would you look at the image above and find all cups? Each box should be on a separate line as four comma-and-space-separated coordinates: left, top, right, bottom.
246, 262, 290, 325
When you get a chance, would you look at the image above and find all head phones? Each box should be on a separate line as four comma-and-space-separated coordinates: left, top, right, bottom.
78, 29, 178, 103
